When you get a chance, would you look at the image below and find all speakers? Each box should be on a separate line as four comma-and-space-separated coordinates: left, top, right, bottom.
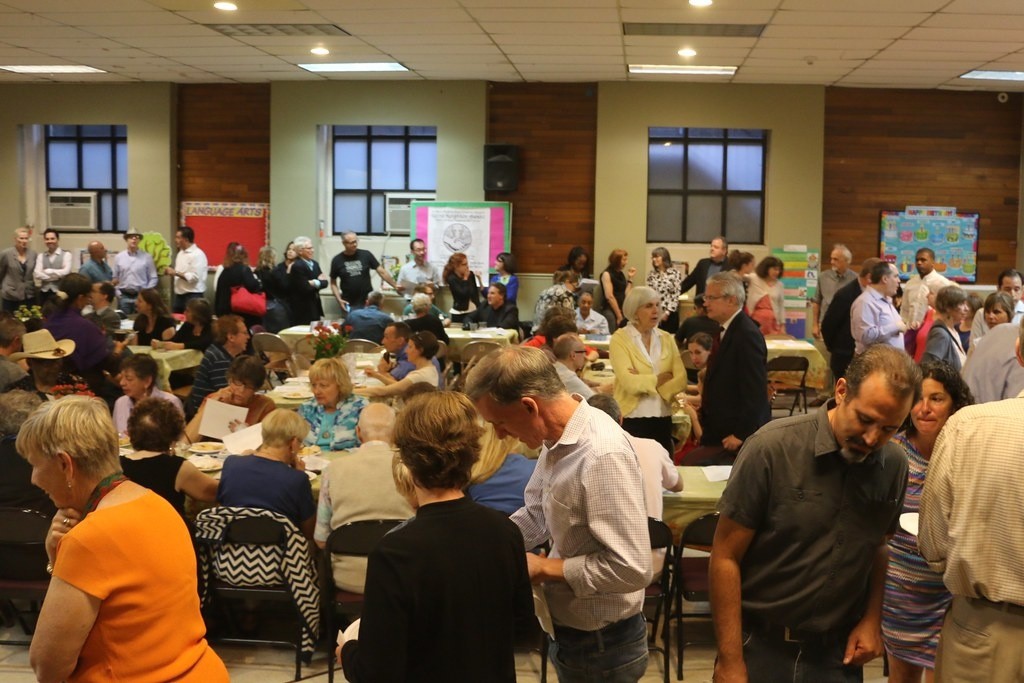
484, 142, 520, 191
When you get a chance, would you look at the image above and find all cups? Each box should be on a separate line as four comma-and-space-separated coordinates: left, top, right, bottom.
470, 323, 478, 333
354, 346, 363, 358
479, 322, 486, 329
350, 375, 365, 386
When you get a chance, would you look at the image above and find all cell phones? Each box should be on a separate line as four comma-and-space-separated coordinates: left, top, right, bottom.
345, 304, 352, 313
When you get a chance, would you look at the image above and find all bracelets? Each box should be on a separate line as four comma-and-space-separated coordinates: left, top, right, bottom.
47, 561, 53, 574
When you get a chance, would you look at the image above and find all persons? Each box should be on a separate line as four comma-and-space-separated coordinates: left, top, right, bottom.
78, 241, 113, 284
880, 361, 975, 683
917, 318, 1024, 683
34, 229, 73, 301
0, 227, 38, 312
0, 230, 1024, 651
464, 344, 653, 683
850, 261, 909, 358
708, 345, 923, 683
608, 285, 687, 460
163, 226, 208, 314
112, 227, 159, 314
701, 272, 771, 466
335, 390, 543, 683
15, 395, 230, 683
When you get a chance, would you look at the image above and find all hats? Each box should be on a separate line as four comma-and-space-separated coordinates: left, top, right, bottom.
124, 226, 144, 241
7, 328, 75, 362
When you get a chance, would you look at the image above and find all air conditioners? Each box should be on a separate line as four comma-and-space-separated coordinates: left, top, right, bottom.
47, 189, 100, 232
382, 192, 435, 234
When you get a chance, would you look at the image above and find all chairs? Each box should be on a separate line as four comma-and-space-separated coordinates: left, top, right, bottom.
0, 299, 809, 683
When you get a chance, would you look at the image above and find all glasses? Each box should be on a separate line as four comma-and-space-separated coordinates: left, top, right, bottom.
415, 331, 424, 345
424, 292, 433, 296
575, 348, 587, 355
231, 376, 258, 391
703, 294, 730, 301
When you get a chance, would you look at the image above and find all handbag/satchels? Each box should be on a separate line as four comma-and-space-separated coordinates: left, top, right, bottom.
230, 285, 268, 315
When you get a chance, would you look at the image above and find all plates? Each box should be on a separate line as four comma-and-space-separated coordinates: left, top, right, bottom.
187, 457, 225, 471
283, 391, 314, 398
188, 442, 226, 454
119, 443, 130, 447
301, 457, 329, 470
304, 471, 317, 481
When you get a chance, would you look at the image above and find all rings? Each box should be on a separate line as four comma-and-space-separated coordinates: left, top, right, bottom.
63, 518, 71, 526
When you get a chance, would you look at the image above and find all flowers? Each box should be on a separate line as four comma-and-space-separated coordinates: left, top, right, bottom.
307, 323, 352, 361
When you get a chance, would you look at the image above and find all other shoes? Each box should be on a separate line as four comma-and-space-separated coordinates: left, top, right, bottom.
808, 397, 825, 406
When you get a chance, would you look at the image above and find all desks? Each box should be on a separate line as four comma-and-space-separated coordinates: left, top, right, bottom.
583, 337, 826, 396
104, 432, 736, 543
126, 343, 202, 401
276, 325, 518, 365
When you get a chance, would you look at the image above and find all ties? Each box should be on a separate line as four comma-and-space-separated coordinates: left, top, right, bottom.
710, 327, 725, 358
